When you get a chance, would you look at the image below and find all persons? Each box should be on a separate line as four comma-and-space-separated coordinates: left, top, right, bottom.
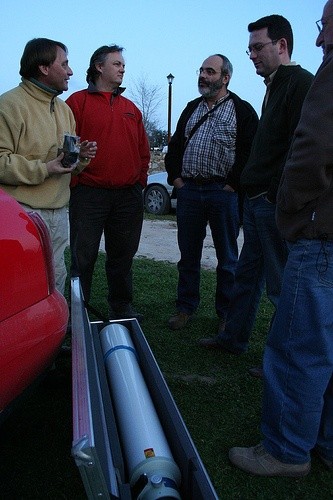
166, 55, 260, 330
64, 44, 151, 317
0, 39, 98, 321
195, 14, 315, 380
226, 0, 333, 476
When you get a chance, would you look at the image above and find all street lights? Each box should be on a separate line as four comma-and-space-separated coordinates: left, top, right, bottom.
166, 73, 175, 144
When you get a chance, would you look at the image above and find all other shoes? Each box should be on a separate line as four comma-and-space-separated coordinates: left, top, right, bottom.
215, 318, 225, 335
53, 345, 72, 357
108, 308, 143, 321
248, 367, 263, 378
312, 449, 333, 468
168, 310, 195, 329
229, 441, 311, 477
199, 337, 248, 355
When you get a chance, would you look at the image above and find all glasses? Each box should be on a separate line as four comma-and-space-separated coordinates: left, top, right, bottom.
246, 39, 278, 55
316, 14, 333, 32
199, 67, 221, 75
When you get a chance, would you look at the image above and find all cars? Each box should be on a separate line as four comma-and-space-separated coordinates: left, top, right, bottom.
142, 171, 179, 215
150, 146, 160, 151
1, 184, 72, 427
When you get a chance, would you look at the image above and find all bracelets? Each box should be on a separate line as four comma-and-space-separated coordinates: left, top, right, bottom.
78, 153, 89, 161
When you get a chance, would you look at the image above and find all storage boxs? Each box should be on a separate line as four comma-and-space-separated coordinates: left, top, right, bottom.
71, 276, 218, 500
